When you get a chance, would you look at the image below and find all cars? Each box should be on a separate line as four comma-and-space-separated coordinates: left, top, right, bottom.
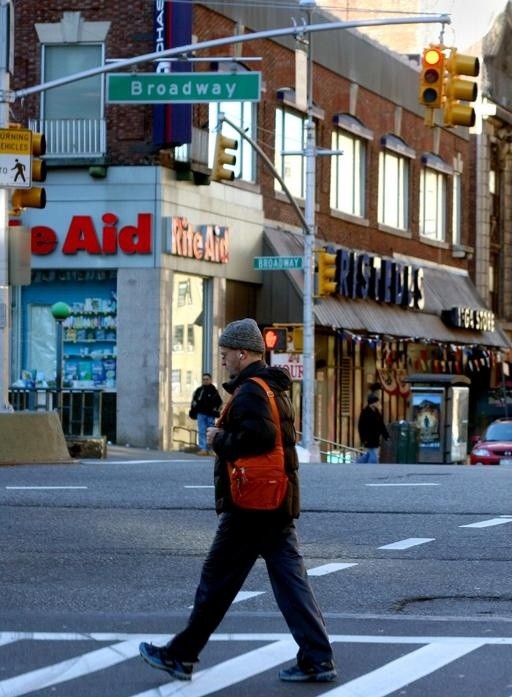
468, 416, 512, 466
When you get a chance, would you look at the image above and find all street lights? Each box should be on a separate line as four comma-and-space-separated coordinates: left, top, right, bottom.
50, 299, 74, 427
208, 131, 242, 187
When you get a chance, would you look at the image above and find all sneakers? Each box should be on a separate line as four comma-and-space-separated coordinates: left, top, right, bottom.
139, 642, 193, 681
279, 660, 337, 683
199, 448, 217, 458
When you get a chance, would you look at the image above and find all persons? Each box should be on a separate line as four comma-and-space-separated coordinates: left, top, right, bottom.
137, 315, 338, 683
357, 393, 392, 463
188, 372, 224, 457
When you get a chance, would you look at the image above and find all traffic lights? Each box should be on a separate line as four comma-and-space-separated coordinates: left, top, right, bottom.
10, 123, 49, 212
263, 325, 289, 353
416, 40, 481, 131
314, 248, 339, 300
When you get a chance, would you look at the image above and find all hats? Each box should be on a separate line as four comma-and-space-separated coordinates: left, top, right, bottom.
219, 318, 266, 354
367, 395, 378, 402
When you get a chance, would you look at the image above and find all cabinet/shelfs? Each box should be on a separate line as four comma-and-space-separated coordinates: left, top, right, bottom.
63, 307, 115, 386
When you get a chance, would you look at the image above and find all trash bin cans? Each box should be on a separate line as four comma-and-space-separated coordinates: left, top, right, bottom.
380, 423, 420, 464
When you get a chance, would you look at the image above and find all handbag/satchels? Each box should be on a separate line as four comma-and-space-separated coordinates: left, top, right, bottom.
190, 402, 202, 417
227, 446, 288, 511
